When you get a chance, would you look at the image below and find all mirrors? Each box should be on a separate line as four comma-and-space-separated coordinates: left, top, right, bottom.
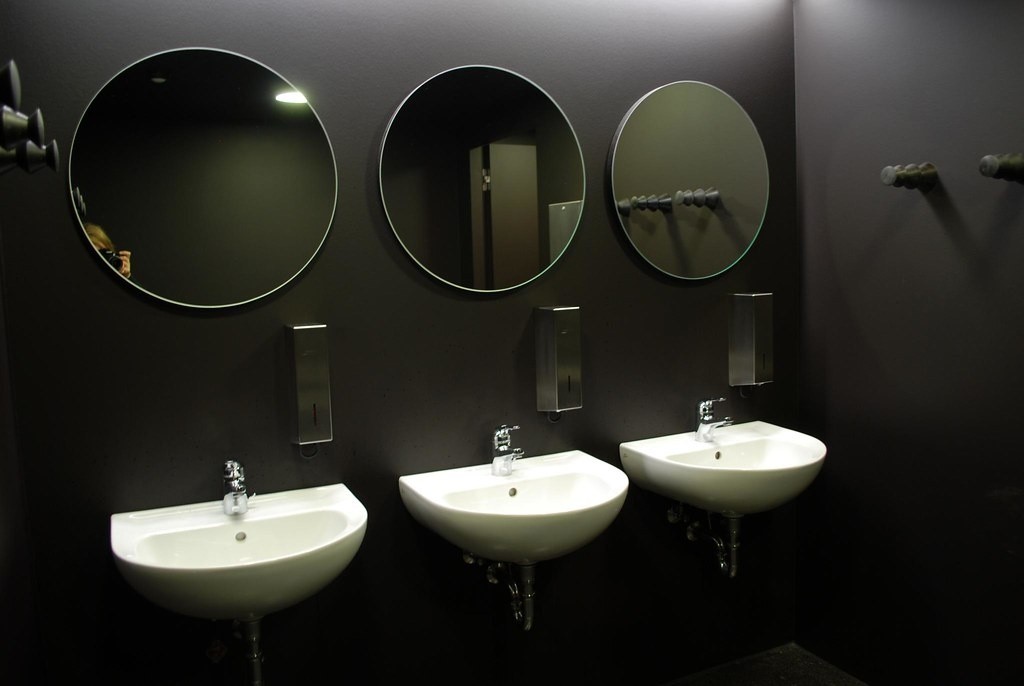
605, 80, 770, 281
67, 47, 338, 309
378, 64, 586, 292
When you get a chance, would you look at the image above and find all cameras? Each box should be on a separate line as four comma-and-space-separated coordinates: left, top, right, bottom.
98, 248, 123, 271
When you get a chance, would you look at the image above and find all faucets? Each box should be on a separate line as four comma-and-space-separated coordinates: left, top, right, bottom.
695, 396, 733, 441
488, 423, 525, 476
222, 459, 251, 518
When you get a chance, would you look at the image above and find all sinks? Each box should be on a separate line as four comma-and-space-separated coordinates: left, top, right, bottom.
397, 448, 631, 566
109, 481, 371, 621
619, 417, 828, 518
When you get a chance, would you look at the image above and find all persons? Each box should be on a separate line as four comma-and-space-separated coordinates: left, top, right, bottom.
84, 222, 139, 285
